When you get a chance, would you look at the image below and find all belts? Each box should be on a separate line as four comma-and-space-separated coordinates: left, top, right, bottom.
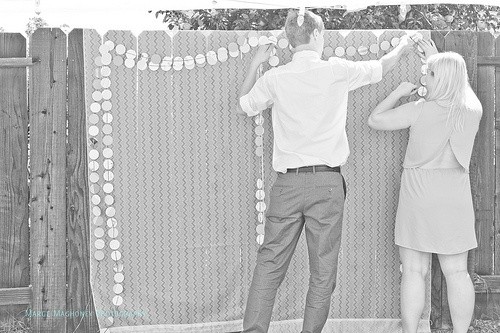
287, 166, 341, 172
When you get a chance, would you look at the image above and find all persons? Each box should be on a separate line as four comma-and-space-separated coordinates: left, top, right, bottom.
368, 36, 484, 333
233, 8, 425, 333
